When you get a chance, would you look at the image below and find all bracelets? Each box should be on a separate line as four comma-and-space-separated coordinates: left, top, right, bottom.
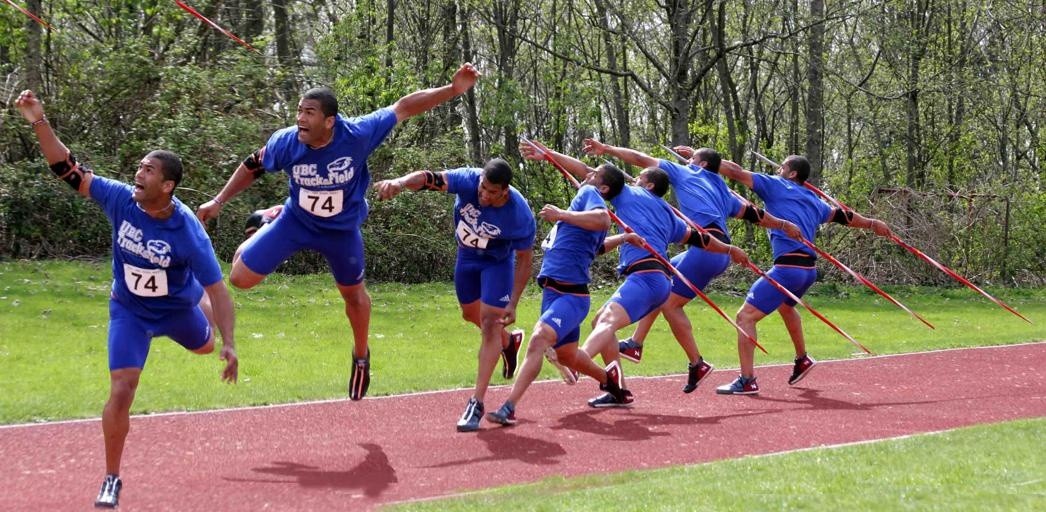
31, 116, 47, 129
213, 198, 224, 207
399, 181, 406, 190
727, 246, 732, 256
868, 219, 873, 228
621, 234, 625, 244
781, 220, 785, 231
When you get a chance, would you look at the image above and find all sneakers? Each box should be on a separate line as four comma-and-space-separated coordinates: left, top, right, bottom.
243, 205, 284, 233
348, 346, 370, 401
95, 475, 123, 506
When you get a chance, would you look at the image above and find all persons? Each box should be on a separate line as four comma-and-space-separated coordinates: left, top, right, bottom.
519, 141, 751, 407
372, 161, 535, 433
487, 164, 624, 427
673, 145, 896, 394
582, 137, 804, 393
15, 87, 237, 506
195, 63, 482, 402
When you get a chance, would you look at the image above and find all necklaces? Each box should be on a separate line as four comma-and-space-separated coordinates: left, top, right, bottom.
309, 128, 335, 150
137, 203, 172, 214
